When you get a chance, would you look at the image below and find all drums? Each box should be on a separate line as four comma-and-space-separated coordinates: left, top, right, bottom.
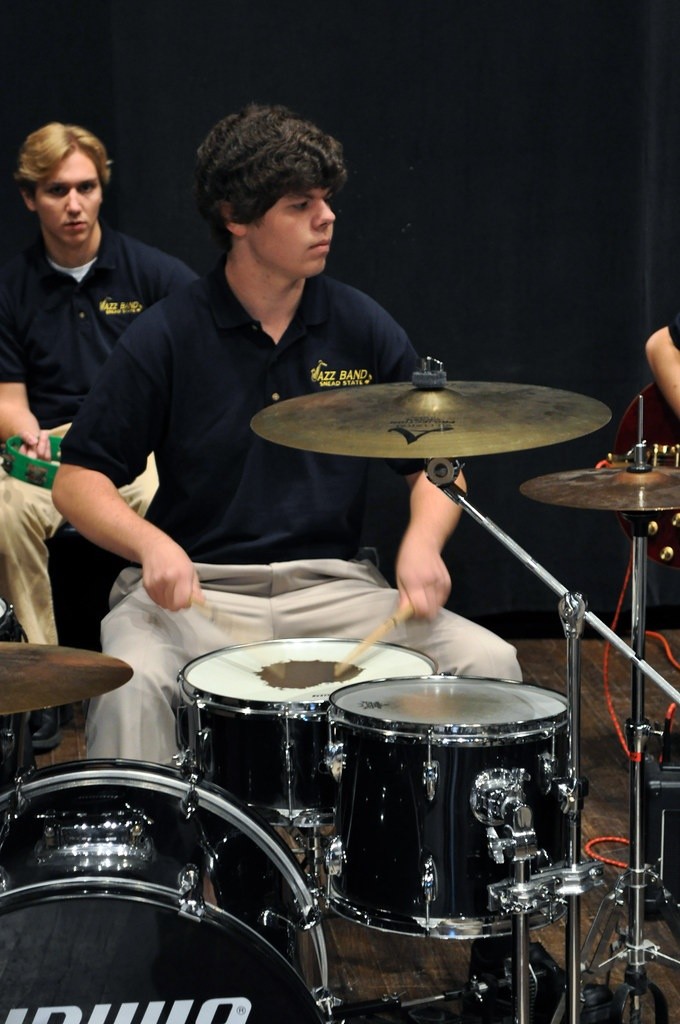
0, 758, 341, 1024
326, 675, 581, 939
177, 637, 434, 878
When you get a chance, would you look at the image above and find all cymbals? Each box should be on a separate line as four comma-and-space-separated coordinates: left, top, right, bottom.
0, 642, 132, 715
0, 433, 63, 491
250, 357, 615, 459
519, 441, 680, 512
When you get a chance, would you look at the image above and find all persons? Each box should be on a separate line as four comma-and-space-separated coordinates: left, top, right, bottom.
0, 123, 202, 753
646, 312, 680, 419
51, 103, 615, 1023
